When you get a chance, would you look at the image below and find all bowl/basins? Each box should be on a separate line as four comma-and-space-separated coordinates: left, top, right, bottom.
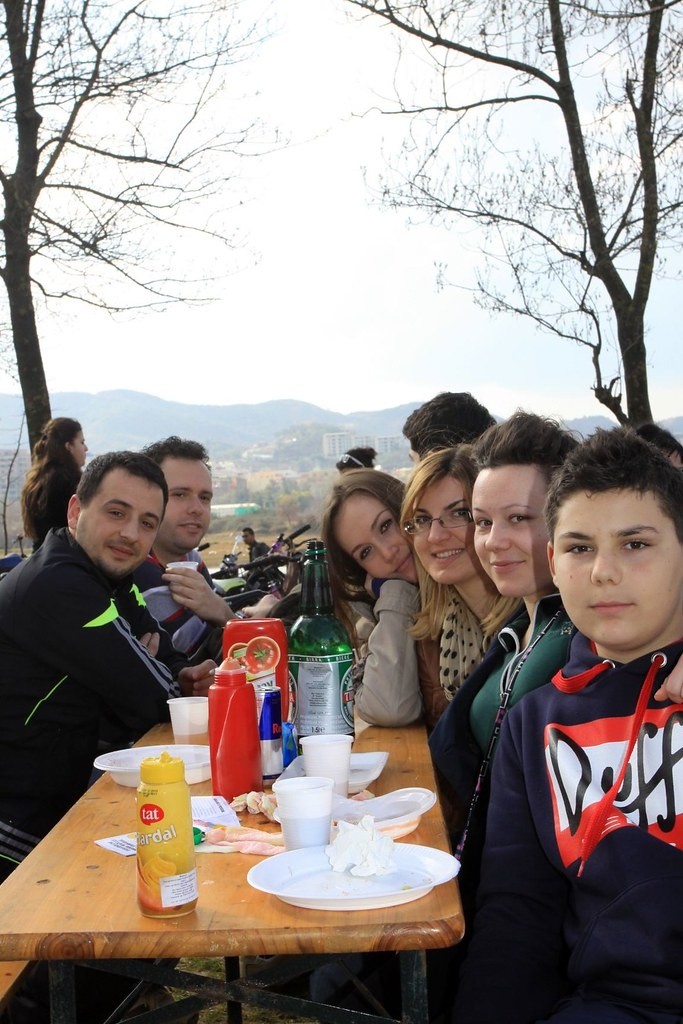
272, 751, 390, 793
273, 786, 437, 841
93, 744, 210, 788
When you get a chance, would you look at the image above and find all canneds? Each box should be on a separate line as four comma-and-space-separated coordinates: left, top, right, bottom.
252, 685, 282, 779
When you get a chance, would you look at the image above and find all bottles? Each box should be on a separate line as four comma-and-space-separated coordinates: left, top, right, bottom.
134, 751, 199, 918
285, 540, 354, 751
208, 658, 263, 806
224, 617, 290, 722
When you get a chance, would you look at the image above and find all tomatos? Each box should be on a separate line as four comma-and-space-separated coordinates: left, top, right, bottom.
229, 638, 279, 674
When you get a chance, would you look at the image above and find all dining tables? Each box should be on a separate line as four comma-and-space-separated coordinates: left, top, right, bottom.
0, 702, 466, 1024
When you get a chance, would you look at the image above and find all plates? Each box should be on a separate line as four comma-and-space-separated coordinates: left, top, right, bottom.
247, 842, 460, 911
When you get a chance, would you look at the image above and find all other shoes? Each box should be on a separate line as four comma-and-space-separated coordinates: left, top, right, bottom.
117, 982, 200, 1024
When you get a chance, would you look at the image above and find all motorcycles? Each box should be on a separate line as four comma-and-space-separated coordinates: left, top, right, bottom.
194, 524, 322, 617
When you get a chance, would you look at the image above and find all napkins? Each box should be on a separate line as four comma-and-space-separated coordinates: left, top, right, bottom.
326, 813, 398, 877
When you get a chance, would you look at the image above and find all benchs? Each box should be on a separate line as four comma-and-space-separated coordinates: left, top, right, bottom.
0, 960, 38, 1016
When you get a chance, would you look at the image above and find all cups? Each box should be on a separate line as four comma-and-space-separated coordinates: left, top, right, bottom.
166, 696, 208, 745
166, 561, 198, 572
299, 733, 354, 800
272, 776, 335, 849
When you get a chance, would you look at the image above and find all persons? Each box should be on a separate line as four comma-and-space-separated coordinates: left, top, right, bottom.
20, 417, 88, 555
0, 452, 218, 1024
321, 390, 683, 1024
139, 438, 281, 668
242, 527, 271, 564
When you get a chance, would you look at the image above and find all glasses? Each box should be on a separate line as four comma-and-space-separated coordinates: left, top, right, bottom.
338, 453, 366, 469
401, 511, 473, 529
